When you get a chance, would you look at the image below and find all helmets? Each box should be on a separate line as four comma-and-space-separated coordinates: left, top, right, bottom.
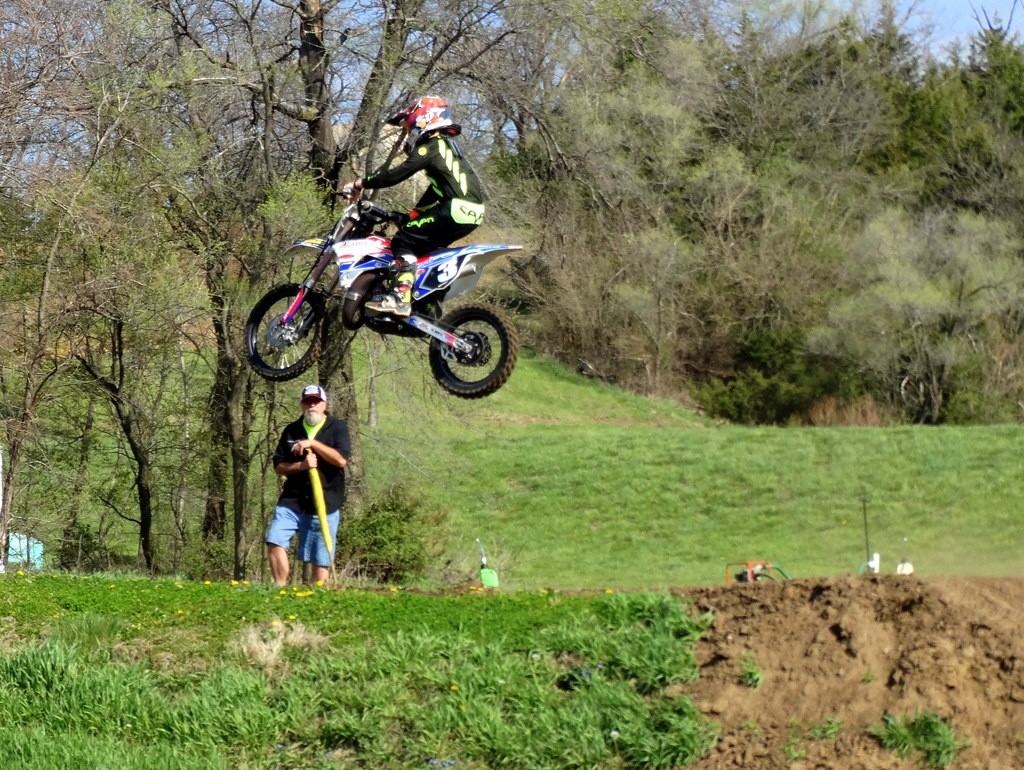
386, 95, 453, 156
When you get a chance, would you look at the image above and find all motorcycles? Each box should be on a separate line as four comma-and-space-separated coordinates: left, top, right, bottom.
242, 188, 524, 399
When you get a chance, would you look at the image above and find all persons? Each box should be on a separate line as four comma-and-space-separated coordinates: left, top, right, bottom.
264, 385, 352, 586
343, 93, 485, 317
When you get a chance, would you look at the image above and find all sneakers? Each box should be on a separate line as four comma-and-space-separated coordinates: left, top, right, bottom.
364, 294, 412, 317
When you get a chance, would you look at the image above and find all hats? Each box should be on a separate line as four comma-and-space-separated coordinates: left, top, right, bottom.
301, 385, 327, 402
